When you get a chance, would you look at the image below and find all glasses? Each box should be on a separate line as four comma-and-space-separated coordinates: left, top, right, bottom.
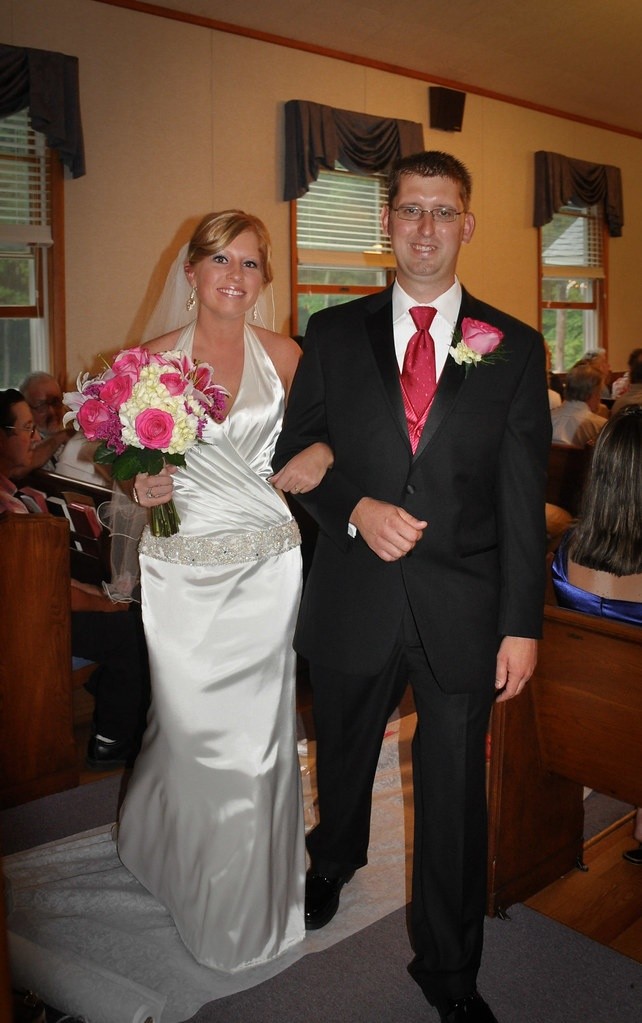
28, 396, 63, 413
2, 424, 37, 439
390, 203, 464, 223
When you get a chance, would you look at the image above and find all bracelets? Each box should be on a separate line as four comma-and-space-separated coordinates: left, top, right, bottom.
132, 488, 138, 504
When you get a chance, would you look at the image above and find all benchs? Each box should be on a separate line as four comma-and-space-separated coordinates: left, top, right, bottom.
0, 400, 641, 920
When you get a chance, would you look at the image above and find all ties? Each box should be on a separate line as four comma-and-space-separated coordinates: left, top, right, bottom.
399, 306, 438, 456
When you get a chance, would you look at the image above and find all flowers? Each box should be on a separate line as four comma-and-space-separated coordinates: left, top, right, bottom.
450, 318, 512, 382
60, 344, 233, 542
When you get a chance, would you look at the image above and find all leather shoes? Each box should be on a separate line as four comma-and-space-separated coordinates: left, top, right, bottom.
305, 865, 351, 930
88, 735, 137, 771
422, 979, 500, 1023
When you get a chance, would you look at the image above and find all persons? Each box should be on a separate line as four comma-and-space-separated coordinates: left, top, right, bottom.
102, 210, 333, 975
551, 365, 608, 448
544, 341, 609, 420
551, 403, 641, 626
612, 348, 642, 399
611, 362, 642, 416
19, 371, 113, 491
0, 389, 142, 768
270, 151, 554, 1023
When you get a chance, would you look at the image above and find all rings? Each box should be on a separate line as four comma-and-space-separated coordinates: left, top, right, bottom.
295, 485, 300, 492
146, 487, 153, 498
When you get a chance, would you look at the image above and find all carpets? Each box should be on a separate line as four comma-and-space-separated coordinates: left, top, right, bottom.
0, 708, 642, 1023
